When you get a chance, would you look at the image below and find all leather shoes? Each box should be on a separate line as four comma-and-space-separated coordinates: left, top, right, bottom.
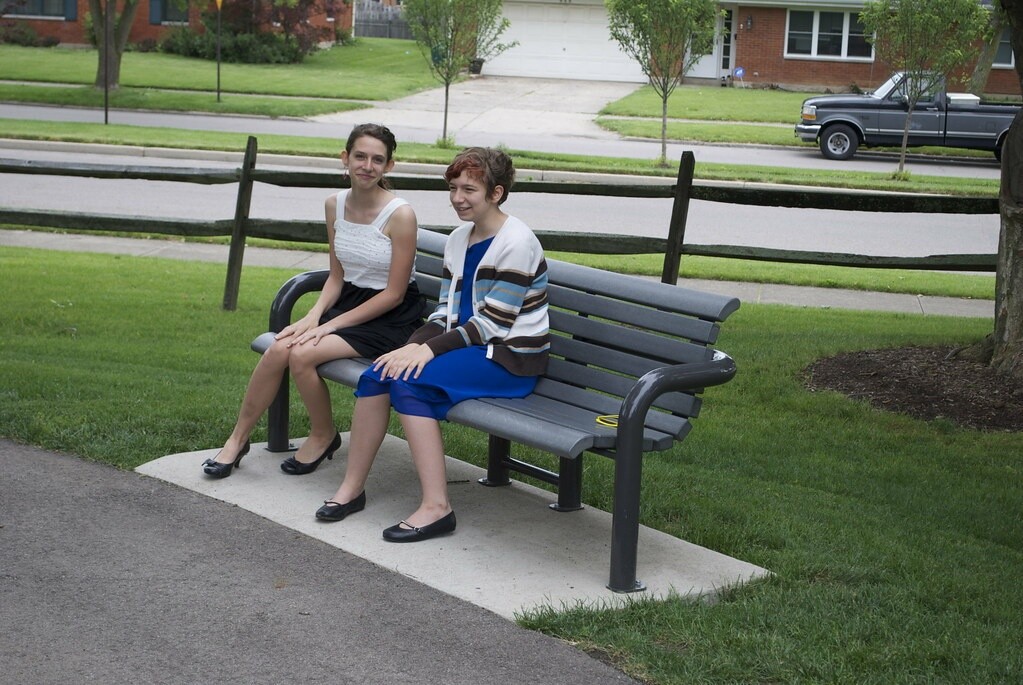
316, 490, 366, 521
383, 511, 456, 542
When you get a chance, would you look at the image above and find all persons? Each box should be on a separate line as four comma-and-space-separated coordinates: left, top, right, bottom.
315, 148, 549, 542
201, 123, 427, 479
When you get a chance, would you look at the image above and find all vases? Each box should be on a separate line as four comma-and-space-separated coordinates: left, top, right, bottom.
467, 57, 484, 74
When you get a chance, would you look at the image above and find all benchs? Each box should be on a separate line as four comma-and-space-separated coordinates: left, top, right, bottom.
250, 228, 741, 594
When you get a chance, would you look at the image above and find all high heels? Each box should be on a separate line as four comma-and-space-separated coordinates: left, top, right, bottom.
281, 431, 342, 475
201, 439, 251, 477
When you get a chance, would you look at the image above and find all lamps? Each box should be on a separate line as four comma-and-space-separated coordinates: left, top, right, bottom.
746, 15, 753, 30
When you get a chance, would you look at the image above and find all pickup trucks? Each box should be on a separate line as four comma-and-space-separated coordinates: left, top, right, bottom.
794, 70, 1023, 163
